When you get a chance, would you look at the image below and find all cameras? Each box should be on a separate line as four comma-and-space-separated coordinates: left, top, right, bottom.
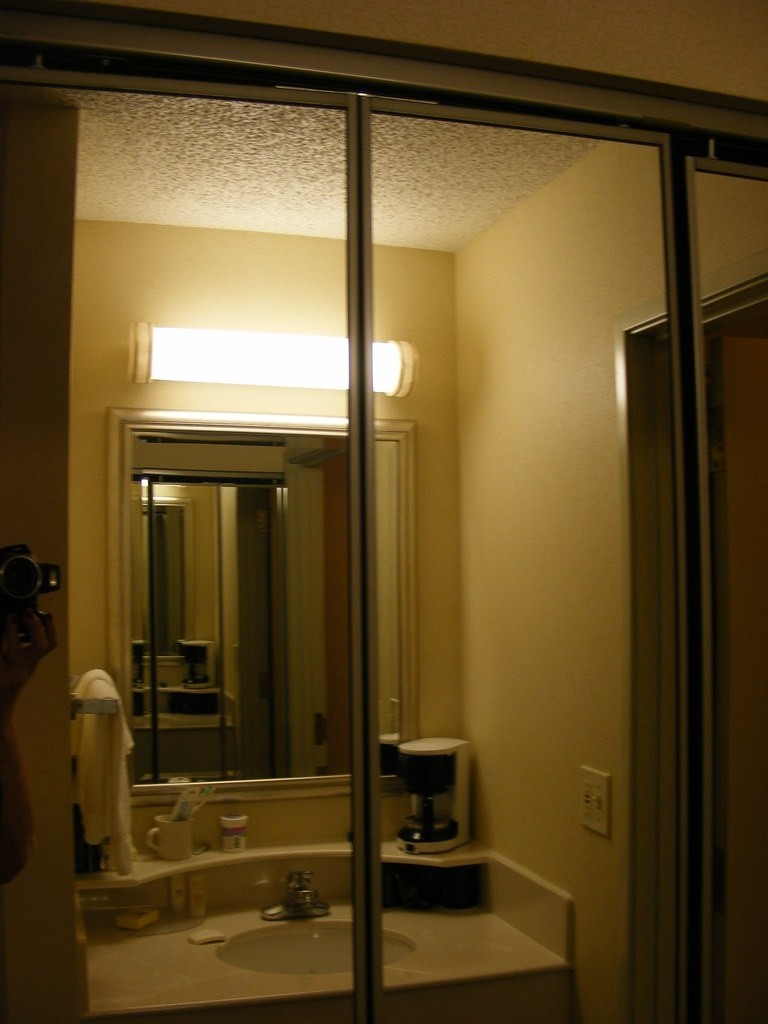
0, 543, 61, 642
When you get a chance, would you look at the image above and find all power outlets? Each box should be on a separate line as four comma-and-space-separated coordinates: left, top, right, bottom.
577, 765, 613, 838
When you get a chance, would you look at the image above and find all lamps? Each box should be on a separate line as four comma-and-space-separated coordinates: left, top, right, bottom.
125, 321, 421, 399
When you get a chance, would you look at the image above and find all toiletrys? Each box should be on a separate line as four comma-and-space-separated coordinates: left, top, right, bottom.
168, 872, 208, 918
218, 811, 250, 854
168, 787, 202, 821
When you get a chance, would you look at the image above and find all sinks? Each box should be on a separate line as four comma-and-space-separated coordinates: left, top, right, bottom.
214, 922, 418, 977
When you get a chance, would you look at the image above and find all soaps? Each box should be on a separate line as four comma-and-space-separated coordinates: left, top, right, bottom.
188, 928, 227, 945
115, 906, 160, 930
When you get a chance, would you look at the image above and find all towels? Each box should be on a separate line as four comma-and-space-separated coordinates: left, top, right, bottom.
69, 667, 139, 877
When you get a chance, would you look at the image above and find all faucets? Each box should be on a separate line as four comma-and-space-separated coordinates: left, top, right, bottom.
259, 867, 330, 922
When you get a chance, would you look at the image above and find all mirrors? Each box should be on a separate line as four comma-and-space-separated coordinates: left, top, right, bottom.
102, 406, 418, 810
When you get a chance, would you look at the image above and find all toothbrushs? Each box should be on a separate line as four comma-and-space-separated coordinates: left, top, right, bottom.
188, 785, 217, 818
